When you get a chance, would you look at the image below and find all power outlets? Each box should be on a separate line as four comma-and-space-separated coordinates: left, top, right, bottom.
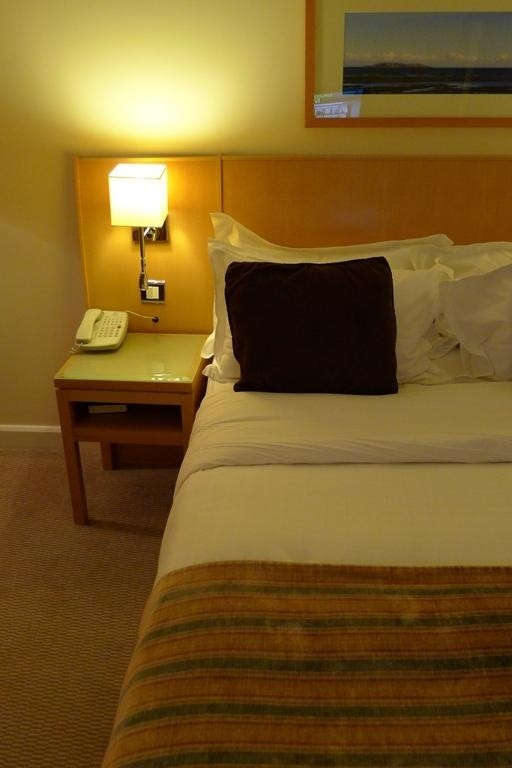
140, 280, 165, 302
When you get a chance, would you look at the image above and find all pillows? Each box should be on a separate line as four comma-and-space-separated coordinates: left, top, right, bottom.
413, 240, 512, 341
200, 209, 459, 358
222, 253, 403, 397
441, 264, 512, 386
202, 244, 452, 387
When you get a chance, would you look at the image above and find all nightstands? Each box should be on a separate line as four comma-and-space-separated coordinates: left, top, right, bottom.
54, 328, 214, 528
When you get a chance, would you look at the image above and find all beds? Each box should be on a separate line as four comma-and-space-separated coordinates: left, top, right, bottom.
96, 381, 512, 768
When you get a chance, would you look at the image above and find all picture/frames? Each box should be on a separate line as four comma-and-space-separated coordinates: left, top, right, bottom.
302, 0, 512, 132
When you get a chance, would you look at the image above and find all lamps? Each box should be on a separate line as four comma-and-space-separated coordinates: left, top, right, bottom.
103, 161, 172, 303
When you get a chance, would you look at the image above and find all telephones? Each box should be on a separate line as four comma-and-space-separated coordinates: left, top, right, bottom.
74, 309, 128, 351
